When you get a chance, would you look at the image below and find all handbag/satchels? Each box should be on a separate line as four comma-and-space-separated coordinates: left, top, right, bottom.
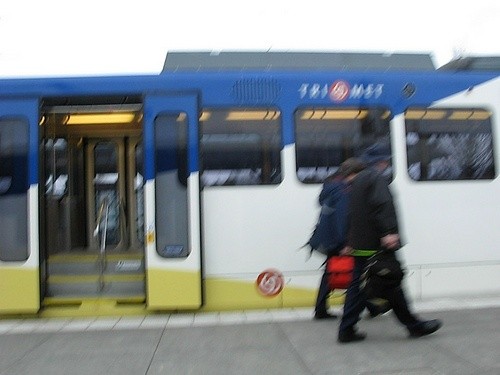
329, 255, 355, 288
361, 242, 403, 305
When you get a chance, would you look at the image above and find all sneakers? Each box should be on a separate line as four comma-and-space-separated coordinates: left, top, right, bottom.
412, 319, 441, 337
339, 333, 366, 342
316, 313, 338, 321
370, 306, 393, 318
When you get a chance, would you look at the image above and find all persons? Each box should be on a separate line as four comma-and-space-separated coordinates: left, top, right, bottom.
300, 144, 441, 344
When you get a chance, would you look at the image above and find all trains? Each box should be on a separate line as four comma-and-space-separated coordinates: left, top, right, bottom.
0, 71, 500, 320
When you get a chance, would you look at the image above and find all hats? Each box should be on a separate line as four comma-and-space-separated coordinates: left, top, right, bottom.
365, 143, 391, 161
